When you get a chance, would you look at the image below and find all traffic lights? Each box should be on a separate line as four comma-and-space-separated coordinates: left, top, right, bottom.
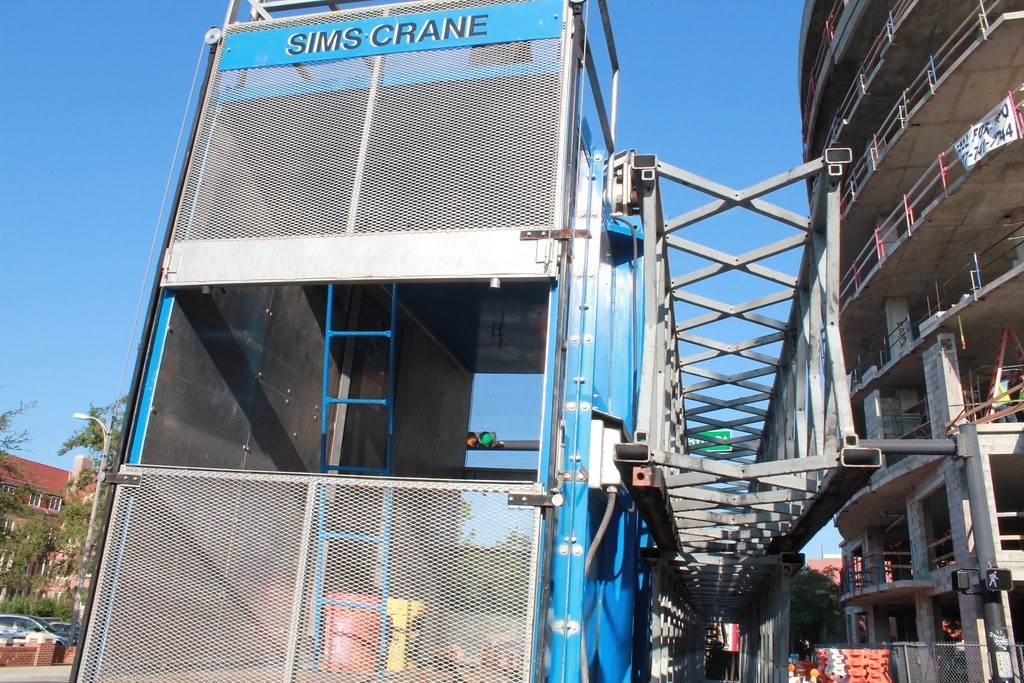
986, 568, 1013, 592
465, 430, 497, 449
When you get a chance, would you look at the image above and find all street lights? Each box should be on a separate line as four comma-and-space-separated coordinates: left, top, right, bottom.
67, 412, 109, 646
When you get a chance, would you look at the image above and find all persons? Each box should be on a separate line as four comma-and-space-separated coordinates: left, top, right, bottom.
799, 649, 812, 664
703, 638, 732, 683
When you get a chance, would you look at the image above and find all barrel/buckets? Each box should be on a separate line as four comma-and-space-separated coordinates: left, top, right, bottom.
383, 597, 426, 674
321, 590, 384, 672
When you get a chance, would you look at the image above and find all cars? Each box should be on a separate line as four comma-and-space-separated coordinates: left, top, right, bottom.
41, 617, 61, 625
50, 622, 79, 645
27, 614, 68, 643
0, 612, 69, 648
